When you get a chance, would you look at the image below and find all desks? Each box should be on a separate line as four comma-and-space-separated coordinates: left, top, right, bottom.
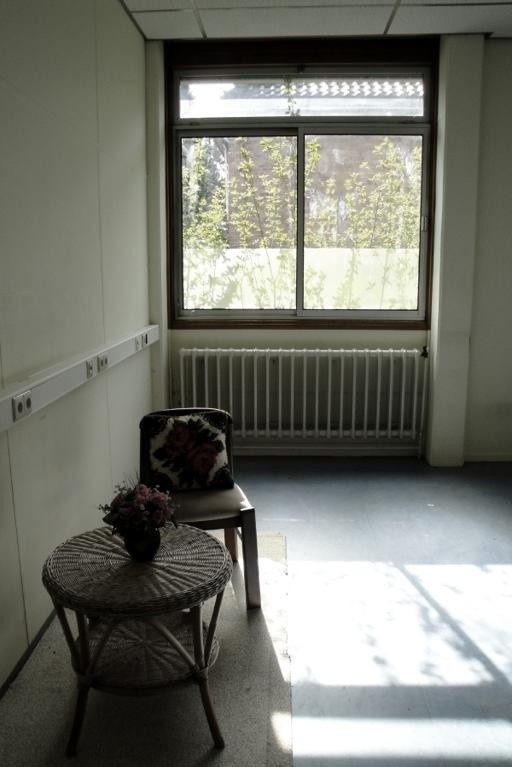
43, 520, 234, 758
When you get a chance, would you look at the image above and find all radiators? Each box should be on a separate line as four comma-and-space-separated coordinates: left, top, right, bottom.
172, 343, 425, 455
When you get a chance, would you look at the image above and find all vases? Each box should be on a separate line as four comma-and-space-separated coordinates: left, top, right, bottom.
124, 529, 161, 562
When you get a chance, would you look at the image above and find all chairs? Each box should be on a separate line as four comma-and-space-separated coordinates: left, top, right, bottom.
139, 406, 268, 612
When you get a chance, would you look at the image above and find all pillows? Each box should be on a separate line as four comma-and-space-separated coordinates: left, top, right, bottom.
140, 411, 238, 491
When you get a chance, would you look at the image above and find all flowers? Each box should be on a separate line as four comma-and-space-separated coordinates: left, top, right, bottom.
98, 474, 183, 531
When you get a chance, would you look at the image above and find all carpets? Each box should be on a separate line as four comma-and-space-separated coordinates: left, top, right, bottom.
6, 527, 296, 767
